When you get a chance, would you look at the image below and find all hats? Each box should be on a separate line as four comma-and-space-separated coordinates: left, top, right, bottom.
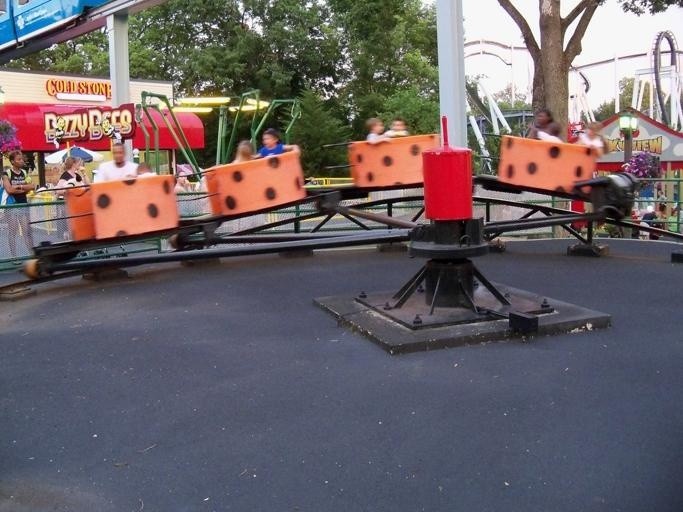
263, 128, 281, 144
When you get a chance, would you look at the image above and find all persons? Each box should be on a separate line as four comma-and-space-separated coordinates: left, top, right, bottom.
365, 118, 392, 145
74, 160, 91, 185
383, 118, 408, 138
174, 170, 193, 193
574, 125, 605, 158
232, 140, 256, 165
98, 142, 139, 182
528, 110, 560, 140
54, 158, 85, 241
251, 127, 302, 159
631, 190, 678, 240
3, 150, 37, 257
126, 163, 157, 179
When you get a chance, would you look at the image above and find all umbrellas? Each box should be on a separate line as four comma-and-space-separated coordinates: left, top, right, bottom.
43, 144, 104, 165
